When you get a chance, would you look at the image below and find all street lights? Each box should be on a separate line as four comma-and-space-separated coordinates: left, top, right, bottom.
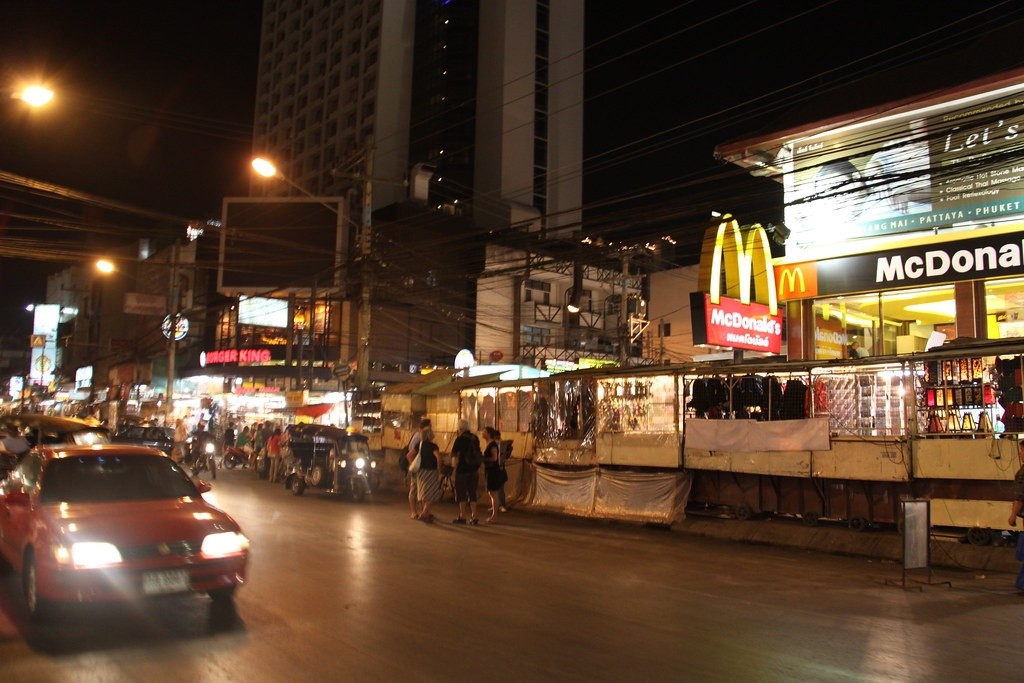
252, 158, 373, 393
96, 259, 178, 429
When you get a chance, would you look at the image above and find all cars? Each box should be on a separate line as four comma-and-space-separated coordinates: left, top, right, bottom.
112, 416, 175, 457
0, 444, 250, 618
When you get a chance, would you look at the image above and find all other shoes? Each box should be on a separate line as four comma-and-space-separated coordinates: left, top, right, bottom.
410, 512, 433, 522
453, 517, 465, 524
468, 518, 478, 525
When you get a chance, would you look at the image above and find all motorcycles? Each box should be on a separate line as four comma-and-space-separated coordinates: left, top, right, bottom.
224, 446, 248, 470
190, 441, 216, 478
0, 413, 111, 478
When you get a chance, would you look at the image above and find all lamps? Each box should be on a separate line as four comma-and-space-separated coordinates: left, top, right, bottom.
924, 358, 998, 435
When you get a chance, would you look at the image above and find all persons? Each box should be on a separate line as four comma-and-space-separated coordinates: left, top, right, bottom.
405, 419, 441, 521
1, 423, 31, 454
451, 418, 482, 525
151, 418, 159, 423
225, 420, 292, 482
849, 342, 869, 359
173, 405, 208, 467
481, 426, 503, 524
814, 118, 932, 223
1007, 440, 1024, 590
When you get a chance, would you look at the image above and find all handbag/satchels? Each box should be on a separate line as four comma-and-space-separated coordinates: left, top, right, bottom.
409, 441, 422, 473
399, 445, 408, 471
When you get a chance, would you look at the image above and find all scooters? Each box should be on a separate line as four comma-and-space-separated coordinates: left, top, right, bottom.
284, 422, 376, 501
